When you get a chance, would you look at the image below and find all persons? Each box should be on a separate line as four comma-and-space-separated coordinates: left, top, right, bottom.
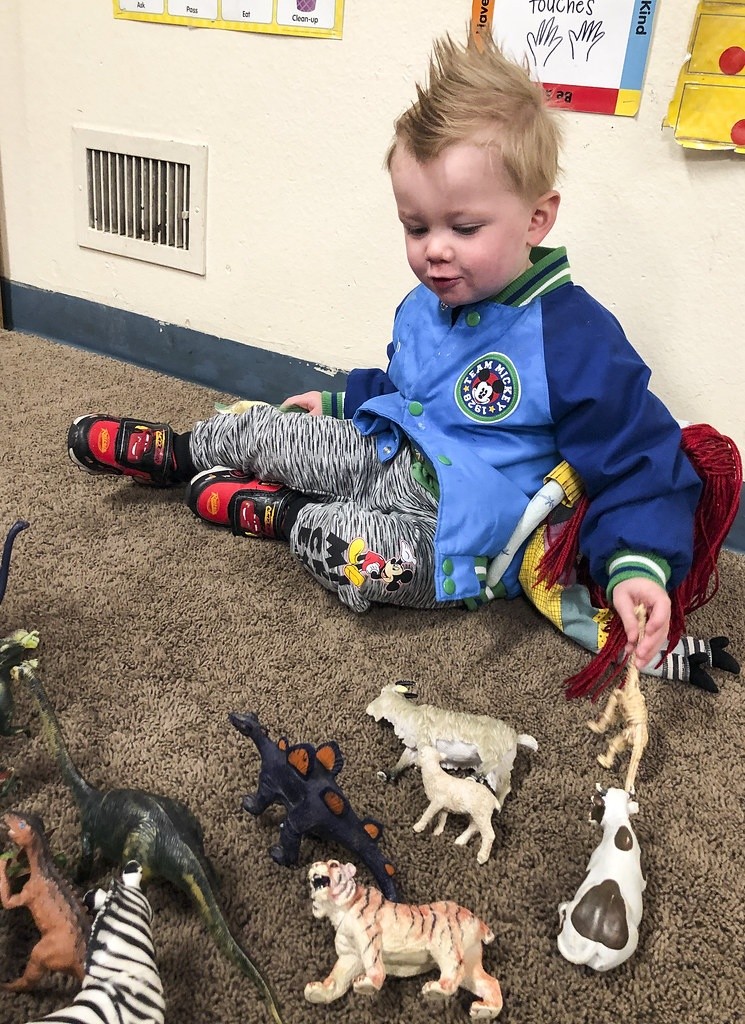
65, 19, 704, 669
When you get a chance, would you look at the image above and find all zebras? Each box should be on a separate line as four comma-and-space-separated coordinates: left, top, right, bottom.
21, 860, 167, 1024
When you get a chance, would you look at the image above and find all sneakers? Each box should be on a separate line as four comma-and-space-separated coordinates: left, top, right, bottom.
186, 465, 302, 540
68, 413, 186, 487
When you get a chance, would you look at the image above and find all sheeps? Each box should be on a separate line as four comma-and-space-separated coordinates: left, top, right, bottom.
366, 678, 538, 865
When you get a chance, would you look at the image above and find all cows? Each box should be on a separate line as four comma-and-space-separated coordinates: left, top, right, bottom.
556, 782, 651, 972
